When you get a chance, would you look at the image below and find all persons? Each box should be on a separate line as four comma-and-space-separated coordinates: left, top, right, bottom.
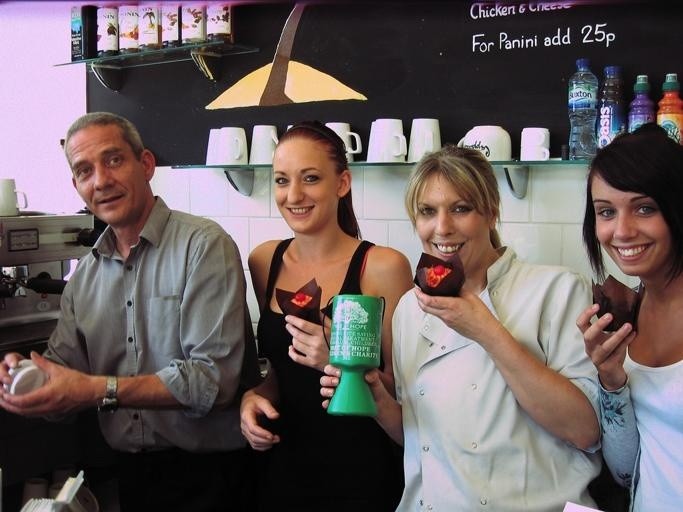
240, 120, 414, 512
321, 147, 598, 512
1, 113, 261, 511
575, 122, 682, 512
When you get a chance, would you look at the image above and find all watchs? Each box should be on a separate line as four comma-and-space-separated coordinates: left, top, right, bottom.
99, 376, 119, 413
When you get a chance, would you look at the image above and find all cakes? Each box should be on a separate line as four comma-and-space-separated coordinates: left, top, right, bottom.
290, 292, 313, 308
423, 264, 452, 288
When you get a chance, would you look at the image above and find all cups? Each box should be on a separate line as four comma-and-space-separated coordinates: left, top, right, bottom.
248, 124, 281, 165
407, 117, 442, 163
0, 178, 29, 218
456, 125, 512, 163
324, 122, 362, 162
367, 118, 407, 163
222, 127, 248, 165
205, 128, 244, 165
519, 126, 551, 162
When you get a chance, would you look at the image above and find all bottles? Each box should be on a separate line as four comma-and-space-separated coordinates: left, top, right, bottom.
137, 3, 161, 50
567, 59, 597, 160
69, 2, 98, 62
628, 75, 654, 134
656, 72, 683, 146
181, 2, 205, 44
595, 66, 626, 149
161, 2, 181, 47
117, 2, 138, 54
95, 3, 119, 57
206, 2, 233, 44
